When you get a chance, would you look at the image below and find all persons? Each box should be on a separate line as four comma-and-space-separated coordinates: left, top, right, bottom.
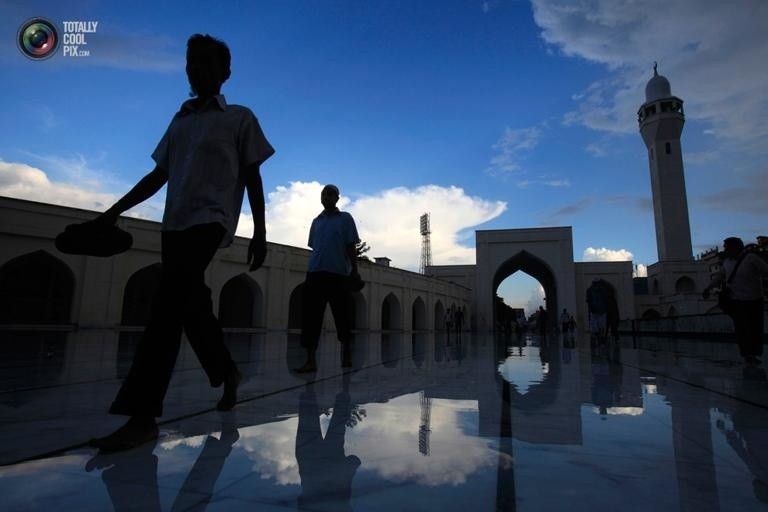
702, 236, 767, 361
443, 306, 578, 337
295, 368, 362, 511
443, 338, 576, 370
291, 183, 366, 374
66, 33, 278, 452
82, 407, 240, 511
714, 362, 768, 503
